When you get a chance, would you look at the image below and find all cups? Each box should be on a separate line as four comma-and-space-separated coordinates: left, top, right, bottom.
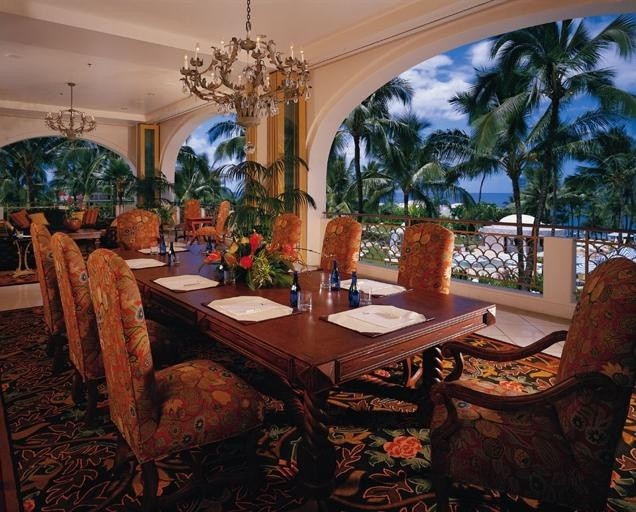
171, 253, 181, 263
297, 290, 313, 312
199, 245, 207, 253
149, 246, 158, 256
319, 271, 332, 288
360, 286, 372, 305
222, 270, 236, 286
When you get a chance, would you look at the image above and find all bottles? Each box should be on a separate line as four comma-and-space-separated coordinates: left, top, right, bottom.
348, 271, 360, 307
330, 260, 341, 291
217, 256, 227, 284
206, 237, 213, 253
159, 235, 166, 255
289, 271, 301, 305
167, 242, 175, 263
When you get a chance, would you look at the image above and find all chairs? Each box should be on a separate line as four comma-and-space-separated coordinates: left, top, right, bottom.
319, 216, 362, 278
50, 229, 164, 426
2, 199, 230, 275
370, 220, 455, 388
427, 255, 635, 510
30, 220, 70, 375
83, 244, 266, 509
269, 212, 303, 265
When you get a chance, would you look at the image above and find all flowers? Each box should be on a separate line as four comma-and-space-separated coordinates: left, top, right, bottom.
200, 228, 307, 289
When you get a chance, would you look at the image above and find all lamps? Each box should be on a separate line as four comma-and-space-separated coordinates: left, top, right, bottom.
179, 0, 312, 128
45, 83, 97, 137
